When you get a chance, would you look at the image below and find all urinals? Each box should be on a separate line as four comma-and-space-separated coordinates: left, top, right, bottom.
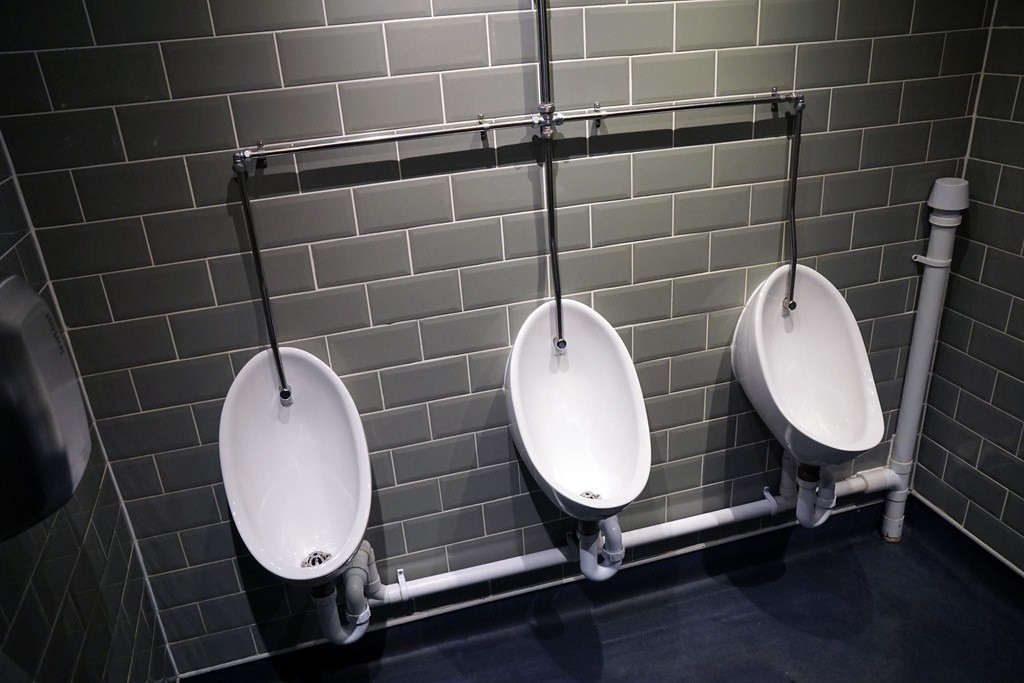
219, 346, 371, 580
731, 263, 885, 467
504, 299, 651, 520
